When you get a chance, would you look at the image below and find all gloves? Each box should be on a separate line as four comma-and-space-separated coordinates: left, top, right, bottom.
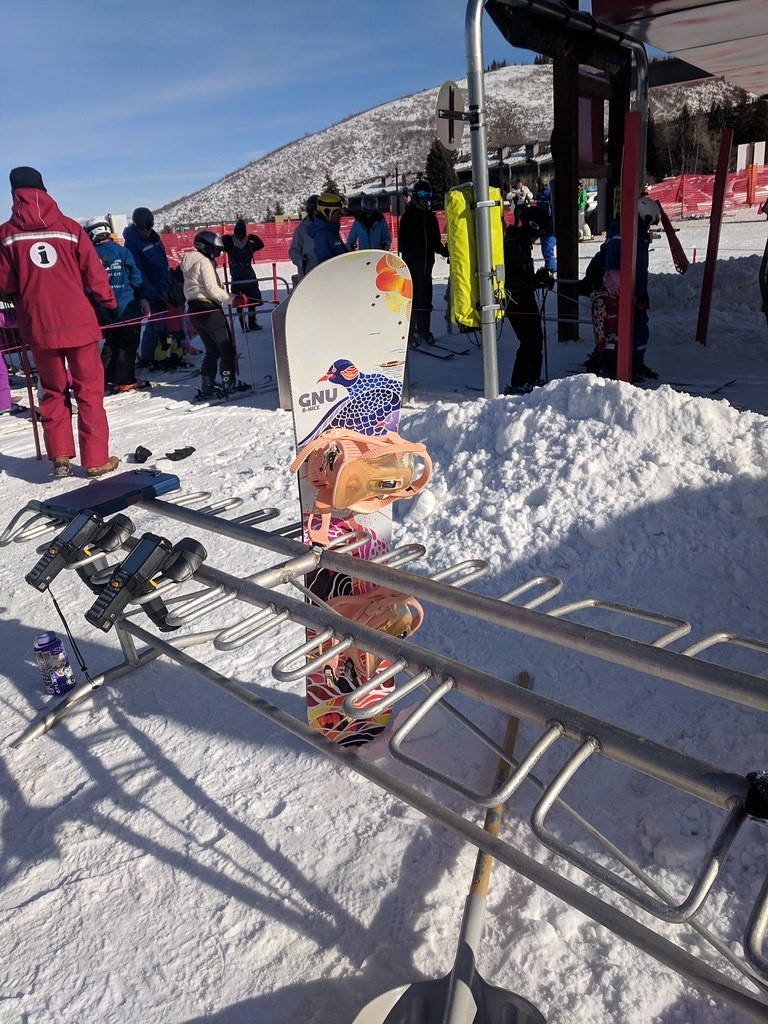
166, 446, 196, 461
135, 445, 152, 463
232, 294, 247, 306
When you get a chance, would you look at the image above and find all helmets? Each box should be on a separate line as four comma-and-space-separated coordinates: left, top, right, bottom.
194, 231, 224, 254
520, 206, 554, 238
361, 194, 379, 213
84, 216, 111, 243
132, 207, 153, 223
537, 175, 550, 186
317, 193, 343, 212
638, 197, 660, 229
307, 195, 320, 210
412, 182, 432, 194
602, 270, 621, 289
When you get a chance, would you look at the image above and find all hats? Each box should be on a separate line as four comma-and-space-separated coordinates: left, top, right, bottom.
9, 167, 46, 193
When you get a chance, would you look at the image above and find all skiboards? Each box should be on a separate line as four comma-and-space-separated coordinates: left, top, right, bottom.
560, 367, 738, 394
99, 367, 201, 396
409, 325, 471, 359
164, 374, 277, 413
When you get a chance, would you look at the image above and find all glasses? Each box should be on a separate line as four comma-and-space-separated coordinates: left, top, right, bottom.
139, 224, 152, 230
417, 190, 432, 199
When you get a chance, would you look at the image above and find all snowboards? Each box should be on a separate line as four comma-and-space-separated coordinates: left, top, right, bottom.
282, 246, 435, 751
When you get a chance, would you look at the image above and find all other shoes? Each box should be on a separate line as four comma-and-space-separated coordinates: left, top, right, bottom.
631, 372, 647, 384
408, 334, 419, 346
0, 404, 26, 416
418, 331, 435, 341
242, 326, 249, 333
636, 361, 654, 376
249, 323, 262, 331
584, 349, 608, 370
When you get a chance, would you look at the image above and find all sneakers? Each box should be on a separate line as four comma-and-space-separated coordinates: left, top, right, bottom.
119, 379, 146, 391
222, 380, 251, 393
155, 359, 176, 369
104, 382, 119, 392
53, 456, 73, 477
533, 378, 547, 387
202, 383, 225, 393
86, 456, 119, 479
512, 382, 535, 393
169, 357, 193, 367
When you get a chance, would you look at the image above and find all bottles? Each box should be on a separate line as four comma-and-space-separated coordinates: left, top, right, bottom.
33, 631, 75, 695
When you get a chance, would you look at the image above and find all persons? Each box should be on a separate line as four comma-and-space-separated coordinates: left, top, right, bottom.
84, 218, 150, 395
181, 231, 251, 400
506, 175, 556, 273
0, 166, 119, 477
289, 193, 349, 281
225, 219, 265, 332
582, 199, 661, 382
124, 207, 204, 369
578, 180, 587, 238
503, 206, 553, 390
347, 195, 392, 251
0, 311, 27, 415
398, 180, 450, 347
